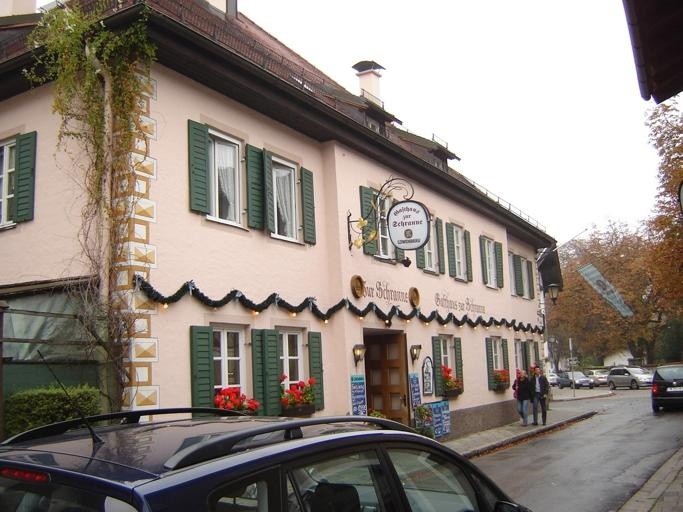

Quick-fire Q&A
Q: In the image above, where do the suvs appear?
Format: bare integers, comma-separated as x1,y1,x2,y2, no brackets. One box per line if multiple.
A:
0,347,536,512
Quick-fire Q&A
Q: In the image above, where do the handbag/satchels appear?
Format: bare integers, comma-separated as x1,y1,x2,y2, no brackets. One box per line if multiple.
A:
513,389,519,399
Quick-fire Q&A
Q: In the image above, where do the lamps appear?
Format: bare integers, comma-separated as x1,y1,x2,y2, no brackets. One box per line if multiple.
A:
545,280,567,306
353,345,367,367
409,345,423,366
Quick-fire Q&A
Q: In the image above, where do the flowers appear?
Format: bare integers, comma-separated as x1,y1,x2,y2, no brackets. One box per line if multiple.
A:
415,403,433,436
441,364,466,391
214,386,261,411
495,368,510,381
277,372,319,405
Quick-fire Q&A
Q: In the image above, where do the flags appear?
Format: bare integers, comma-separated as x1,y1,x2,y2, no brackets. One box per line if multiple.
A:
578,262,634,320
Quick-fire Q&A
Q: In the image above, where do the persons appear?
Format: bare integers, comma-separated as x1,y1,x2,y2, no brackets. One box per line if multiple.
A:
512,370,531,428
528,365,550,426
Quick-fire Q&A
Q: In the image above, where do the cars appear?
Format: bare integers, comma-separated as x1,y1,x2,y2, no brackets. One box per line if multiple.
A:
549,366,654,390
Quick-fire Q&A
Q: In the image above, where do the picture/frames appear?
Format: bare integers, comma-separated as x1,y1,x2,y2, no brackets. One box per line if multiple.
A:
421,356,434,396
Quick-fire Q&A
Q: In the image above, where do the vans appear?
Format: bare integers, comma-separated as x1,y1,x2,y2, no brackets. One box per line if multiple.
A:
651,364,683,414
